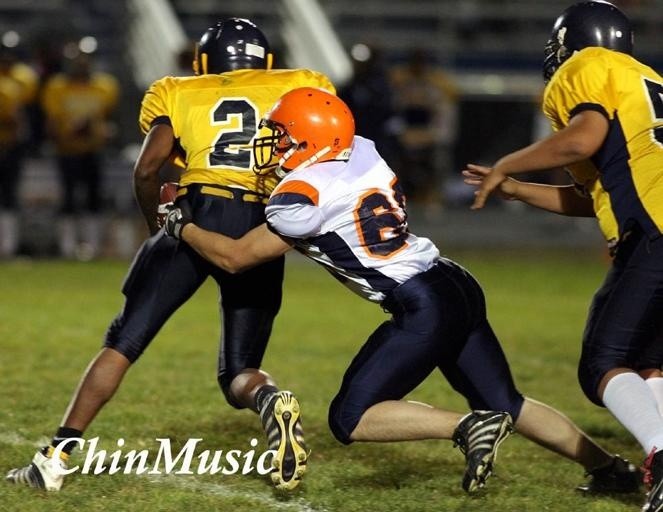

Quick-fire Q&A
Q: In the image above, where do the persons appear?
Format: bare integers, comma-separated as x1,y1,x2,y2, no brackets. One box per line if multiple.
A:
4,16,339,491
459,1,662,511
159,86,649,495
0,27,459,262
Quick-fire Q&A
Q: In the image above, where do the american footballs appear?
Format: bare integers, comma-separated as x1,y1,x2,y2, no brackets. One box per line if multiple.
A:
156,181,178,232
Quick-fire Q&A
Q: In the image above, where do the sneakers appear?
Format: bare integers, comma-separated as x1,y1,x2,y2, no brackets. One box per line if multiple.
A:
452,406,519,493
573,453,639,499
5,449,71,493
638,452,662,512
261,389,312,494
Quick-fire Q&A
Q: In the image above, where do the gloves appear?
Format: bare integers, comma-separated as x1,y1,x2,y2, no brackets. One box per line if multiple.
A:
165,202,193,241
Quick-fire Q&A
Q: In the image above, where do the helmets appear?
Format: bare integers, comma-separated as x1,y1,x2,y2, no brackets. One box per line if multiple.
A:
252,86,356,179
191,15,274,75
541,0,634,86
